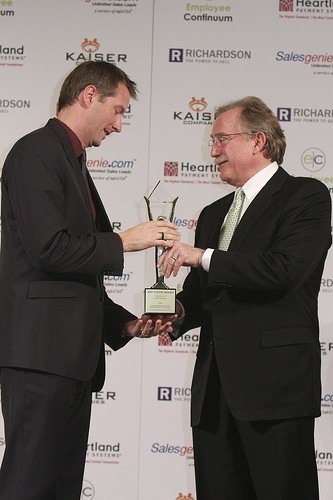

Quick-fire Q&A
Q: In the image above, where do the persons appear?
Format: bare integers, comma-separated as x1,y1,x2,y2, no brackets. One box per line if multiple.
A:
0,61,180,500
142,96,333,500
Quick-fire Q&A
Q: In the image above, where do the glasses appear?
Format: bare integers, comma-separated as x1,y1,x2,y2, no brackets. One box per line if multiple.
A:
209,131,257,147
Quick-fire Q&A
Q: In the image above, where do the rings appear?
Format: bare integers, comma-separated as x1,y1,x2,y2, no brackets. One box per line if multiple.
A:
142,331,148,336
162,232,164,240
172,257,176,260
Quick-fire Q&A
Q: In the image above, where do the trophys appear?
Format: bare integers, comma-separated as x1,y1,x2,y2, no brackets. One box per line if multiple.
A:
143,180,179,315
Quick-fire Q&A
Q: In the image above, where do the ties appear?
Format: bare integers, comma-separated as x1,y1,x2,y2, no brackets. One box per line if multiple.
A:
218,188,246,252
79,150,87,184
